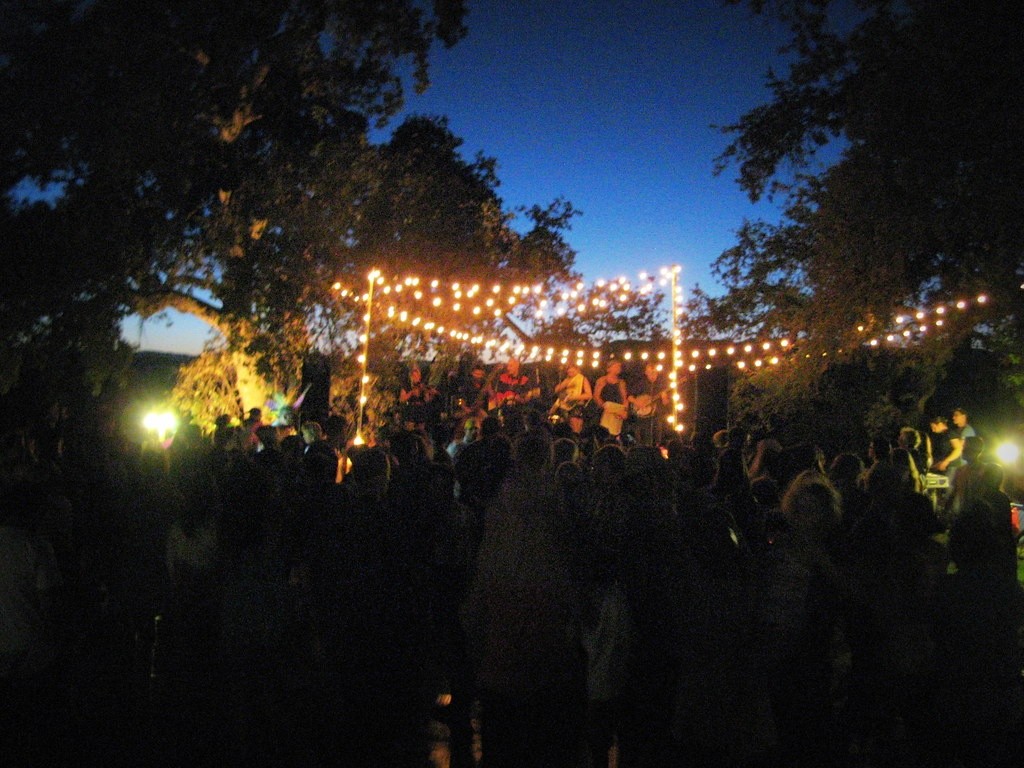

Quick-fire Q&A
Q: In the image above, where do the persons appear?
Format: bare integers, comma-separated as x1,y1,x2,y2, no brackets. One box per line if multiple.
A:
2,320,1024,768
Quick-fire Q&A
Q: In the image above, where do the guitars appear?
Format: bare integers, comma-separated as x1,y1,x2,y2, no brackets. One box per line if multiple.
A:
631,374,688,421
